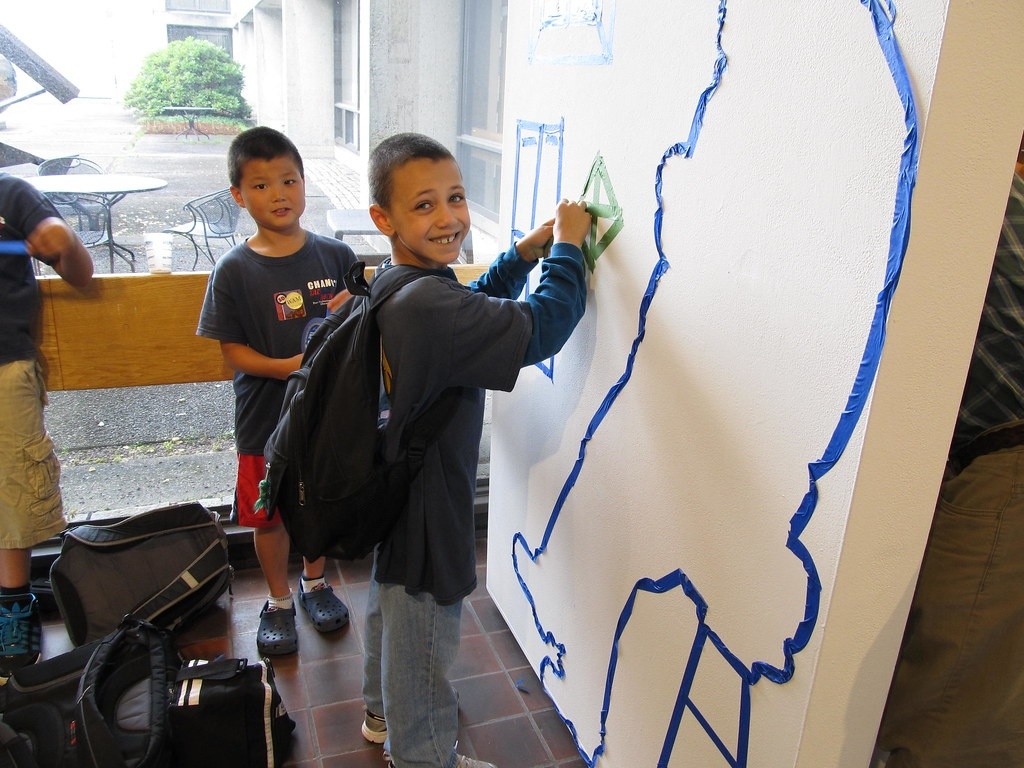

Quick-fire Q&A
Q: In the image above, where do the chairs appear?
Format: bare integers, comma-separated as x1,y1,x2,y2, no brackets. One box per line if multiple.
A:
161,188,242,271
37,192,117,274
37,157,108,232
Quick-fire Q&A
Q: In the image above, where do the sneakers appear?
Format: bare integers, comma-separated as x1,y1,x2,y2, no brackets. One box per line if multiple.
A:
383,740,498,768
0,593,43,686
360,685,461,744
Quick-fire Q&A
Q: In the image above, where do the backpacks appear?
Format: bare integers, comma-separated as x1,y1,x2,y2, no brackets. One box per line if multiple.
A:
49,498,235,635
253,260,433,562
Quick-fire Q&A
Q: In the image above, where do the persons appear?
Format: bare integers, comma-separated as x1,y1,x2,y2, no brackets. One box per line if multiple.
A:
0,173,93,686
196,126,359,654
362,134,592,768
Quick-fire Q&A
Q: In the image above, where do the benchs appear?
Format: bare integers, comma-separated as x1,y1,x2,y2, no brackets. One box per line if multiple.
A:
326,208,473,265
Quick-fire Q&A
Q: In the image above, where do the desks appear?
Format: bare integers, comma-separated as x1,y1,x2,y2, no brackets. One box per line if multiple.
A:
15,174,168,273
163,107,217,141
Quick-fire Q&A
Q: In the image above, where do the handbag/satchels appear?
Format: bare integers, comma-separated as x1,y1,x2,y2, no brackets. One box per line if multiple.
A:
0,624,249,768
163,650,296,768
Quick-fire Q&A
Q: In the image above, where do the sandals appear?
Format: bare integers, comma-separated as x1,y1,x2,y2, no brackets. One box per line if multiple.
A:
255,600,299,655
296,576,350,633
